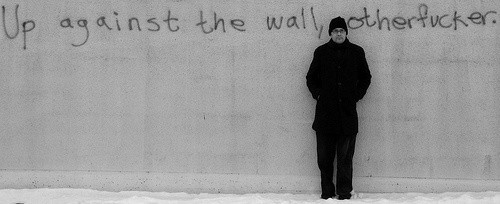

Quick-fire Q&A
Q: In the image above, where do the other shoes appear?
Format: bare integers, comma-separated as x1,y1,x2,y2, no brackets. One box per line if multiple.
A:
319,194,335,199
338,193,351,201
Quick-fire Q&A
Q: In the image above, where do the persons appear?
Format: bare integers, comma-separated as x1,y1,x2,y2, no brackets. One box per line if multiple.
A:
305,16,373,200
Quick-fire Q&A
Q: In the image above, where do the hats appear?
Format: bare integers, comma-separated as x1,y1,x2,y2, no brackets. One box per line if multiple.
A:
328,16,348,35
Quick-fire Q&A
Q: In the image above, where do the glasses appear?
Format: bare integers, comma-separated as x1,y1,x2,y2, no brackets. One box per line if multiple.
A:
331,29,346,34
